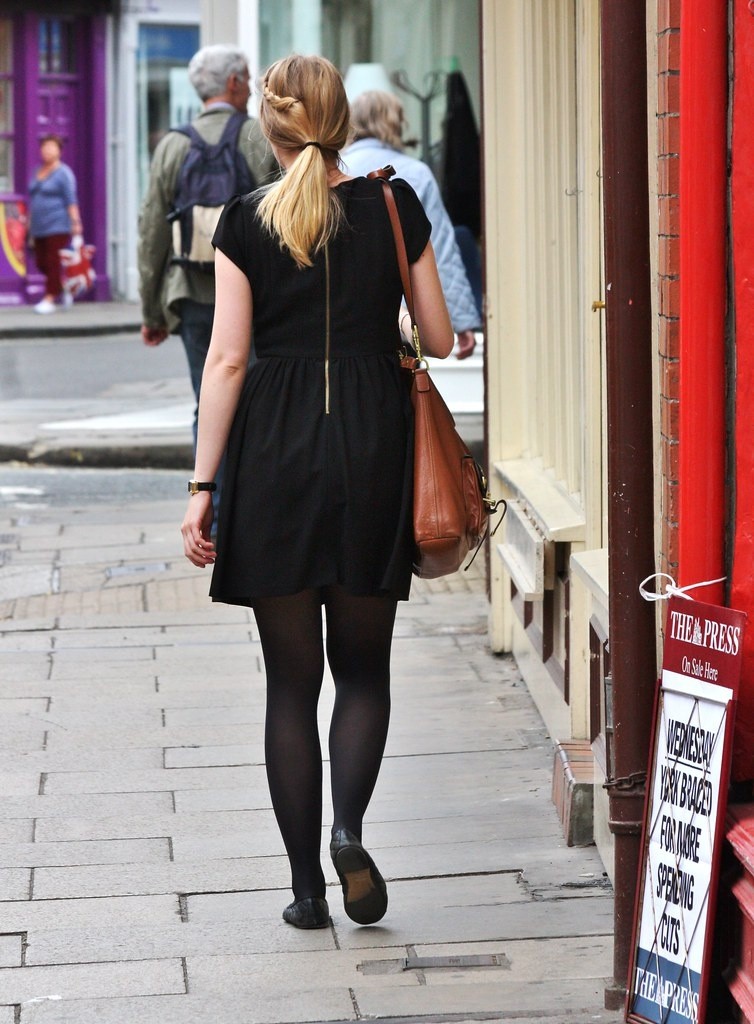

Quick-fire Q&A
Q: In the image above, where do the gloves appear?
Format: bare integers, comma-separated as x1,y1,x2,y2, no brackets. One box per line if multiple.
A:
70,236,84,250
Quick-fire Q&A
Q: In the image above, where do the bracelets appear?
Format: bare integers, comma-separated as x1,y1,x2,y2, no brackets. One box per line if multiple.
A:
187,480,217,496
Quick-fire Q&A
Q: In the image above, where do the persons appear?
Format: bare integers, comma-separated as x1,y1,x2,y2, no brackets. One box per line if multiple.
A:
133,43,287,551
28,133,83,314
337,90,483,361
180,55,455,929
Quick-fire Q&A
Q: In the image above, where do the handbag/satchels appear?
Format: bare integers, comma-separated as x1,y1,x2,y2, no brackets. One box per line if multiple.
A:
368,165,508,579
58,243,98,298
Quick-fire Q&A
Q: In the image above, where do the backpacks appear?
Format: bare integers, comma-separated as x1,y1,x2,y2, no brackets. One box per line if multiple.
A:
170,112,258,272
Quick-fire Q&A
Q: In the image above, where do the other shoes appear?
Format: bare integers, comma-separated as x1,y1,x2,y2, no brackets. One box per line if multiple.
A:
32,300,55,315
283,895,329,929
329,828,388,924
62,293,74,310
